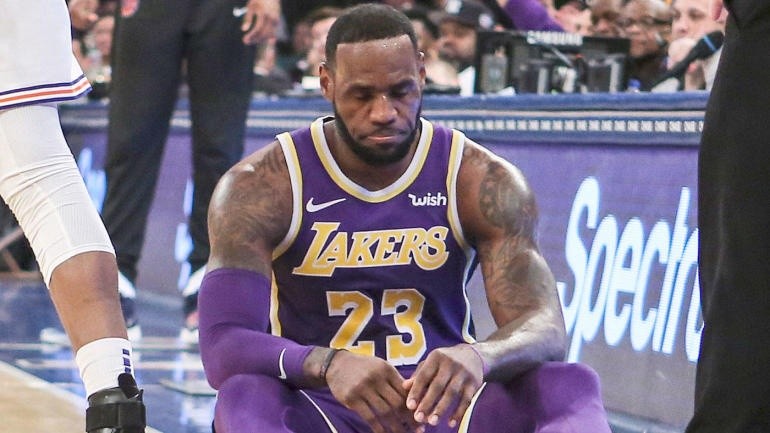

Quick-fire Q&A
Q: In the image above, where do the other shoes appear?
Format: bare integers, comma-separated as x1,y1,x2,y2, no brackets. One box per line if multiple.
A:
85,373,146,433
178,300,201,341
39,290,142,341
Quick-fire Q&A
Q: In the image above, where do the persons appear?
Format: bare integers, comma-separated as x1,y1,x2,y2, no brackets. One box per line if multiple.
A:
197,5,615,432
685,0,770,433
40,0,282,353
68,0,730,97
0,0,148,433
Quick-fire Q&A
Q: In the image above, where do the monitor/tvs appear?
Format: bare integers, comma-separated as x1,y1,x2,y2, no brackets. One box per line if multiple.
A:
473,31,631,95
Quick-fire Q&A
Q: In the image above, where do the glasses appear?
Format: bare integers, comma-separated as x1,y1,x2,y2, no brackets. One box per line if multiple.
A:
620,15,672,30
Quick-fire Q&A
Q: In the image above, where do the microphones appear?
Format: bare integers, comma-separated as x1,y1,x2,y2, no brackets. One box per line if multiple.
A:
648,31,724,91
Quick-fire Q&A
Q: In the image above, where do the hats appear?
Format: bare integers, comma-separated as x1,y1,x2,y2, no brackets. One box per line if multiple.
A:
427,0,494,33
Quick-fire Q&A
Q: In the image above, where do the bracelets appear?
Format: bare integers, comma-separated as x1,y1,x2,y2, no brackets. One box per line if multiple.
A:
318,349,349,384
469,345,490,377
